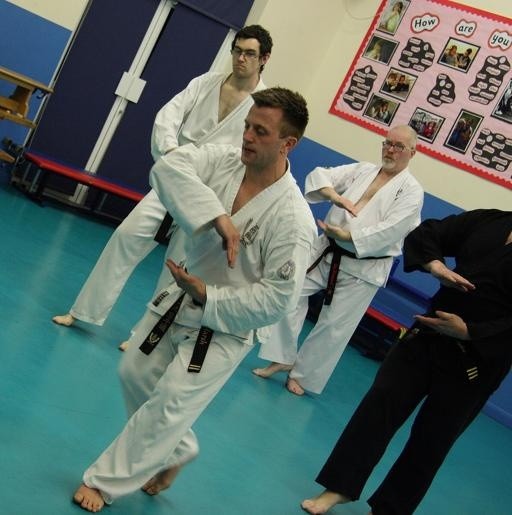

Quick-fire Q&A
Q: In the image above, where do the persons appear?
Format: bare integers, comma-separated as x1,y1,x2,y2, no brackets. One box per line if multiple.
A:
366,41,381,61
300,207,512,513
382,72,410,96
411,120,435,138
448,119,475,149
52,25,272,352
366,100,390,121
71,89,319,513
254,126,424,395
377,2,404,33
444,44,472,70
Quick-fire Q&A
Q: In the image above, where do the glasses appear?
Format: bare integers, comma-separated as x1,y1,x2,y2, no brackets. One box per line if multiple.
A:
383,141,405,154
230,48,261,59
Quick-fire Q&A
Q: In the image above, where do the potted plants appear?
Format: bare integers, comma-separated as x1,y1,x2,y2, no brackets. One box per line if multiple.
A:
0,137,23,164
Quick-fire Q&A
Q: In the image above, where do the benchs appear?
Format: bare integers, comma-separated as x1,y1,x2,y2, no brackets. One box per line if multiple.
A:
11,147,146,226
355,307,410,360
